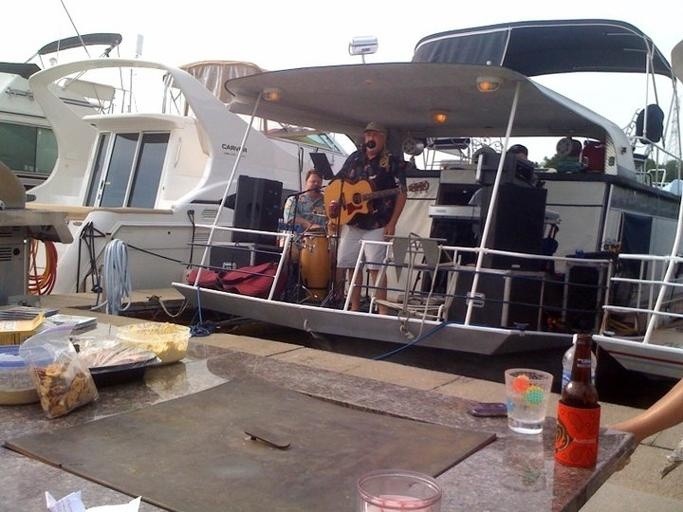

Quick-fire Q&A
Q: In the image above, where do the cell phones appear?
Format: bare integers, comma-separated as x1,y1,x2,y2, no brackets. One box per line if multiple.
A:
470,403,507,417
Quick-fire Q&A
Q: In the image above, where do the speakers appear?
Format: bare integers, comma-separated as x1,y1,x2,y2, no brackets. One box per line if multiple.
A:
230,175,282,246
475,185,548,271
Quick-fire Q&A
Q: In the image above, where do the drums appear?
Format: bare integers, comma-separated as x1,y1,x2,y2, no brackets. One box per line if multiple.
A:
299,231,331,302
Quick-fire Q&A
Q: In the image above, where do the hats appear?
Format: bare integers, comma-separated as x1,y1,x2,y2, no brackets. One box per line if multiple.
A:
362,121,388,133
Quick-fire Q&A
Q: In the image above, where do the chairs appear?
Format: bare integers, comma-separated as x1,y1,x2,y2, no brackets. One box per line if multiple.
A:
425,135,470,170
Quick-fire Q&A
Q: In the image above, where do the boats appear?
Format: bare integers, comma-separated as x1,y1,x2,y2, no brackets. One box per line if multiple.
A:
591,40,682,386
0,32,143,294
170,17,682,377
23,36,377,296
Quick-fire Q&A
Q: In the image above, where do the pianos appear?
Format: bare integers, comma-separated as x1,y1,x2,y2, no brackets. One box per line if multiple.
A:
427,205,561,225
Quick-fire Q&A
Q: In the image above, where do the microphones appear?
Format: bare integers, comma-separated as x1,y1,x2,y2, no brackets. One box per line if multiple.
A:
356,141,376,148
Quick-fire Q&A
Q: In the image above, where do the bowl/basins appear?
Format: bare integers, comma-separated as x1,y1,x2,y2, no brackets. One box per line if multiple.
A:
114,321,192,368
0,345,55,406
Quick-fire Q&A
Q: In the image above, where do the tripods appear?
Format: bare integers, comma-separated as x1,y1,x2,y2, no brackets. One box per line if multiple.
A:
319,154,370,310
287,245,316,302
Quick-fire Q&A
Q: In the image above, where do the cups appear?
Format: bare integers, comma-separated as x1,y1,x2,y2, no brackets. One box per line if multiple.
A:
356,468,442,512
504,367,553,436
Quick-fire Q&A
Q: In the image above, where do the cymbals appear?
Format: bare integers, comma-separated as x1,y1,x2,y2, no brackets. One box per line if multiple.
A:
304,212,328,218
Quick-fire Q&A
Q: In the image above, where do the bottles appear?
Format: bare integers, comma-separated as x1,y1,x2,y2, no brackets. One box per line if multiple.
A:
554,331,600,470
560,332,597,401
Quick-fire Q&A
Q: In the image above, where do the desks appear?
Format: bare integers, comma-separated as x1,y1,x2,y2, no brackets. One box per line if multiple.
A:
0,309,642,511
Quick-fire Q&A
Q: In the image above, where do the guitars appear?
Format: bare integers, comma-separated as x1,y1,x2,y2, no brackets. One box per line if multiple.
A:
324,178,429,226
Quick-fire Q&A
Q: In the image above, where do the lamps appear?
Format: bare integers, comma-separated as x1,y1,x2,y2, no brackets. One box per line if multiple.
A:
346,37,379,64
260,84,284,102
431,110,449,125
475,76,503,94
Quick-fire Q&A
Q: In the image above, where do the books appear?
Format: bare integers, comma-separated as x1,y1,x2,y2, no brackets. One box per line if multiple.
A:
46,315,97,330
0,305,58,321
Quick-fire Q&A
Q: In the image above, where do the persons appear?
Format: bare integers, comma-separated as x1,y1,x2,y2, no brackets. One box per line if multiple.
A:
326,120,408,314
556,129,581,174
506,144,528,161
278,169,330,265
605,379,682,443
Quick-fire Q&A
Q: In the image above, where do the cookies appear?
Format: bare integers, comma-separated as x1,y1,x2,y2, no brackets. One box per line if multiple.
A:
31,363,95,419
